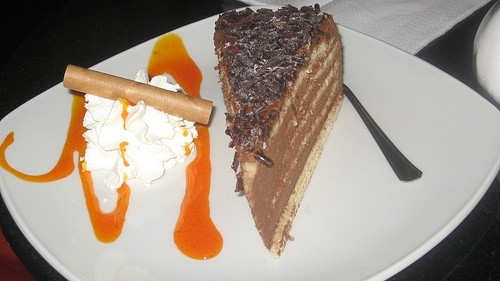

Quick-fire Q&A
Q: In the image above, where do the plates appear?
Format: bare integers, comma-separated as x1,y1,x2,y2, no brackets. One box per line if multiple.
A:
0,5,496,281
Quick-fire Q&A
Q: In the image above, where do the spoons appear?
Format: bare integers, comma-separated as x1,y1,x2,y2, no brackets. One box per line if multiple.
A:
342,83,423,183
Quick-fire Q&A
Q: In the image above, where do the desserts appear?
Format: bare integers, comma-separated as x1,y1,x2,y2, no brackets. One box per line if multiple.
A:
213,4,345,256
